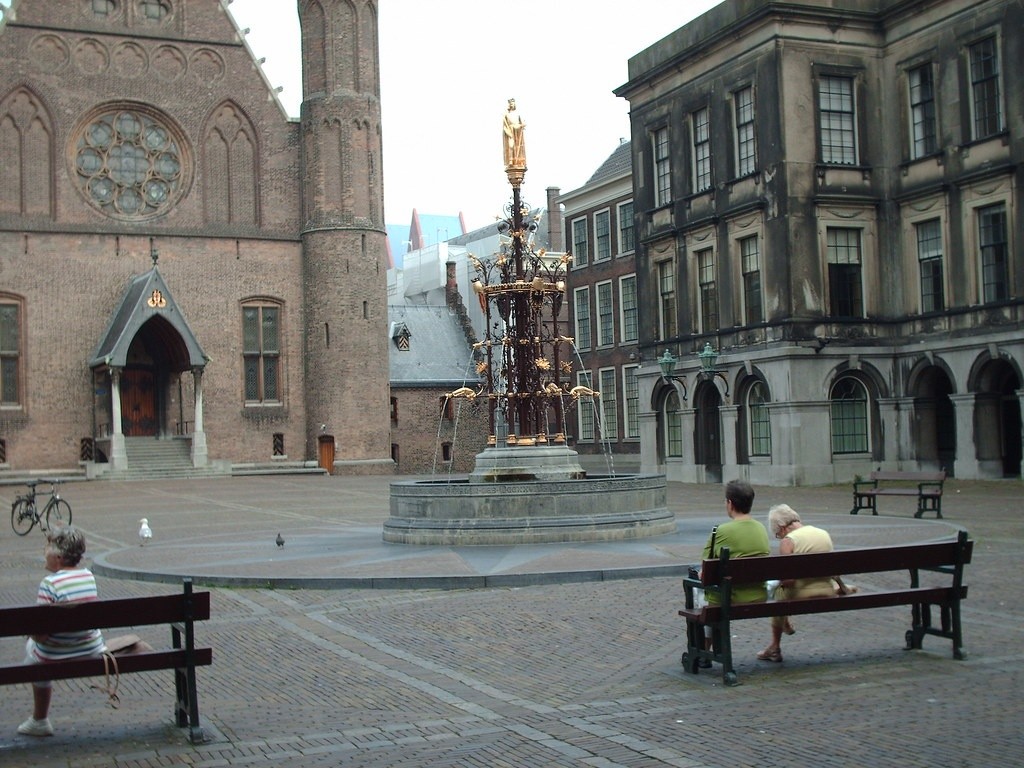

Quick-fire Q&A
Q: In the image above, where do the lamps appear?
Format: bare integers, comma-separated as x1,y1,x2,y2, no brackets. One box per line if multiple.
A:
698,341,730,400
659,349,688,401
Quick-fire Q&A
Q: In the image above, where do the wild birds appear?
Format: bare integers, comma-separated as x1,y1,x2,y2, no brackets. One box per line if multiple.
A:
138,517,153,547
275,532,286,550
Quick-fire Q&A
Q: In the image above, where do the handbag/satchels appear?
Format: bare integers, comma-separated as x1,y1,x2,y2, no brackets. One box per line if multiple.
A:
688,566,699,581
100,633,142,656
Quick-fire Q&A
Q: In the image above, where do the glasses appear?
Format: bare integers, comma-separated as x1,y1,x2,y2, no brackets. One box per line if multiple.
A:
775,533,782,539
722,498,728,503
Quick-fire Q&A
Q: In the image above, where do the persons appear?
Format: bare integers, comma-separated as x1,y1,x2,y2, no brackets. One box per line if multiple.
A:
502,99,526,167
691,479,770,668
17,526,104,736
757,503,836,662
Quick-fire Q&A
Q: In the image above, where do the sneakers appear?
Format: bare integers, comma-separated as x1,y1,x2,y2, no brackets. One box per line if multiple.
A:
16,716,54,736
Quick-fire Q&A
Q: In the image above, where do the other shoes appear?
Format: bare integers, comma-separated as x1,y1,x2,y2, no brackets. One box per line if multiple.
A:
699,657,713,668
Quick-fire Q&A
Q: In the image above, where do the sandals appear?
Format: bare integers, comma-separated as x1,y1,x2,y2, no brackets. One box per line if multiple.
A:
783,621,796,636
756,644,783,662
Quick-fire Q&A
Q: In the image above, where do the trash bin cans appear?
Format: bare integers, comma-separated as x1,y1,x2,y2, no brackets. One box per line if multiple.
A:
318,435,334,474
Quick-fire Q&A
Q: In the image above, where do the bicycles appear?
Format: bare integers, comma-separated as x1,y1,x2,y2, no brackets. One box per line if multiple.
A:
11,478,72,536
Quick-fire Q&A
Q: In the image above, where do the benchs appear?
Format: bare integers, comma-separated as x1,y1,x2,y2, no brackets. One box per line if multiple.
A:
850,466,947,519
678,528,973,687
0,578,213,745
